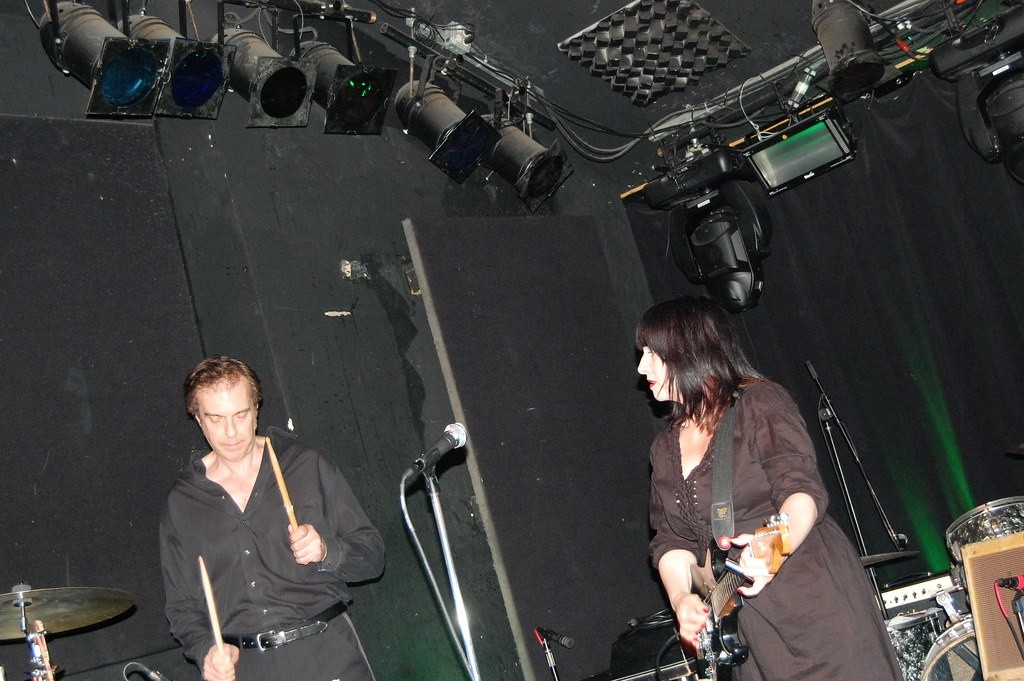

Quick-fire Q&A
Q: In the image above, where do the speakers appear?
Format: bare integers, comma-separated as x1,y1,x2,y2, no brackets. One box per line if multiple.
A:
961,531,1024,681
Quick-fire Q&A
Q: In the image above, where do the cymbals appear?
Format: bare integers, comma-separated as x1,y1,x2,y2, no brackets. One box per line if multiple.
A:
860,550,922,567
0,586,137,640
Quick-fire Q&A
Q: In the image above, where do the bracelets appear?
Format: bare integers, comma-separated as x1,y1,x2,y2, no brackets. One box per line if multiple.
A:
317,539,326,563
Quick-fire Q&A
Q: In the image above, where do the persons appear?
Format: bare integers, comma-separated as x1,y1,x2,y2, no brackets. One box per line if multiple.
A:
635,296,903,681
157,356,385,681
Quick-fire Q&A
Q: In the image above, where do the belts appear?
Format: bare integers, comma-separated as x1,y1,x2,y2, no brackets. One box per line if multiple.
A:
224,600,348,651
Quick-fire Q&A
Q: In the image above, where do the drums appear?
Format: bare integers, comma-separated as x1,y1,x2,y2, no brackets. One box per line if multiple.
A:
884,607,945,681
945,495,1024,605
920,618,985,681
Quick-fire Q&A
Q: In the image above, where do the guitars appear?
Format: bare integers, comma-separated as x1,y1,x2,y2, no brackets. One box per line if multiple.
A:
689,512,791,681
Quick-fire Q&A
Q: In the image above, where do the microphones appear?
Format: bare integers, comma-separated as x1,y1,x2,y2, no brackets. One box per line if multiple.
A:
402,423,469,479
138,662,169,681
995,575,1024,591
898,534,908,552
536,626,575,648
